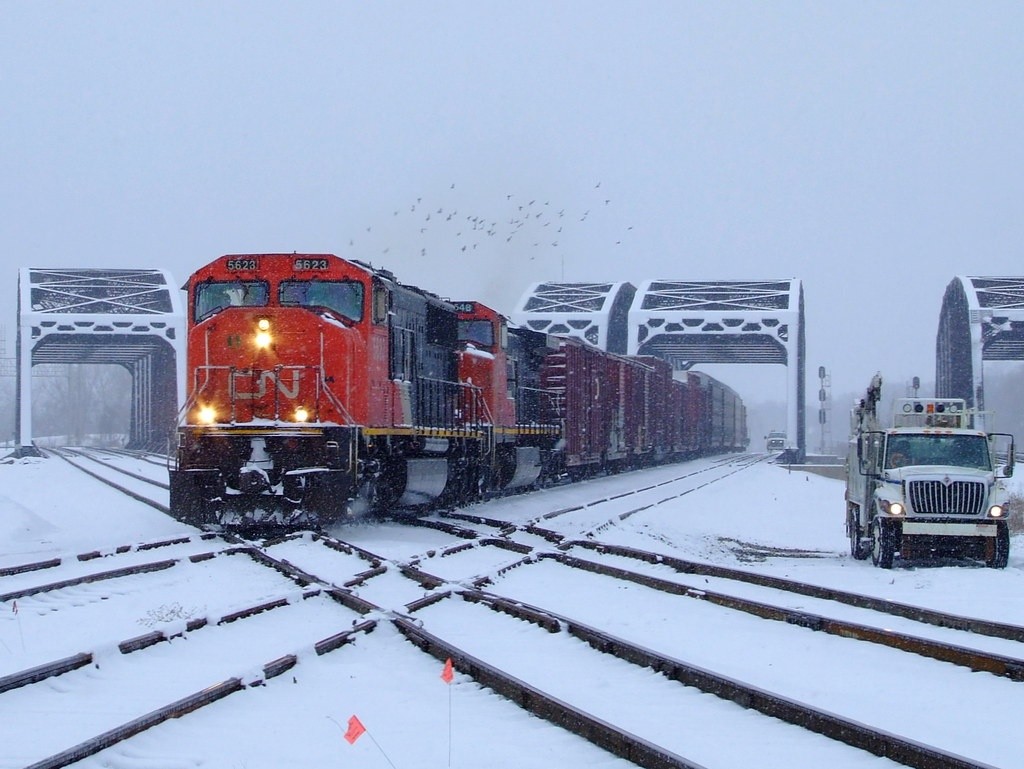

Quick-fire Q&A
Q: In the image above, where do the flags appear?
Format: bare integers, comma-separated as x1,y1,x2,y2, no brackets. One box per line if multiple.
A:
440,659,453,683
344,716,365,745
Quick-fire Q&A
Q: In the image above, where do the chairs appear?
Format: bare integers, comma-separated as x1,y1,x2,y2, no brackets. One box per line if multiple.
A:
890,441,915,468
950,438,974,465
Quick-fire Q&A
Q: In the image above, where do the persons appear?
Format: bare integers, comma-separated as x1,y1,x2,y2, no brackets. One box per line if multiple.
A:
217,293,231,307
888,441,918,467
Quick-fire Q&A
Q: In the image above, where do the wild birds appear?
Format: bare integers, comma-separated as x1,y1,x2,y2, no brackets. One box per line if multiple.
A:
349,182,633,261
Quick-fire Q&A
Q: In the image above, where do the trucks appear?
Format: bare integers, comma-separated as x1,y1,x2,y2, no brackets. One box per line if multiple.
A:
764,430,788,453
843,398,1017,572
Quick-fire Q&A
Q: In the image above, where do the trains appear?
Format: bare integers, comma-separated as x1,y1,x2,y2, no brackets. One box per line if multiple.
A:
168,251,750,543
